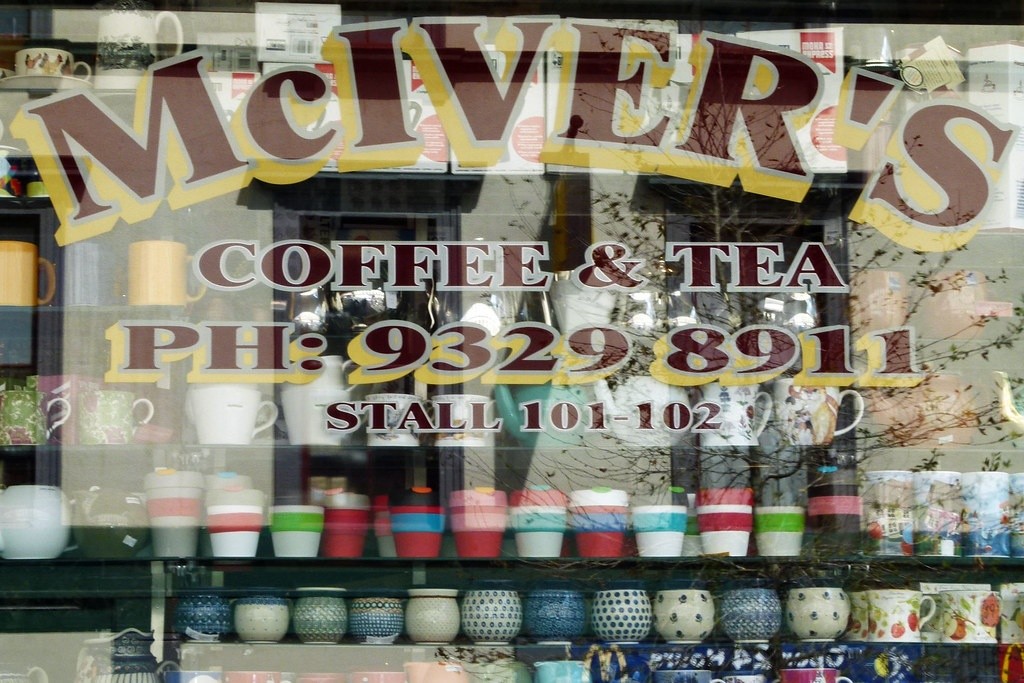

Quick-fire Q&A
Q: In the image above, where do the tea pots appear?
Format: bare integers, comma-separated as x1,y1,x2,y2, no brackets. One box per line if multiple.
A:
493,376,692,448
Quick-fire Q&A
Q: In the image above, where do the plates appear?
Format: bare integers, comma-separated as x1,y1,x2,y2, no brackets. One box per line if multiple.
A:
0,74,94,90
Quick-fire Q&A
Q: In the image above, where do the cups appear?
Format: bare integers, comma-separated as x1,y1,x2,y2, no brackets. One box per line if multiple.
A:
162,660,855,683
0,11,184,90
698,379,865,446
0,240,208,305
0,468,1024,557
0,145,61,197
177,587,1024,642
849,271,991,339
550,270,618,337
0,355,493,447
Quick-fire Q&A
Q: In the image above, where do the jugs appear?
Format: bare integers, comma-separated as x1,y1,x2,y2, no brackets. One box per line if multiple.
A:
865,369,1024,446
